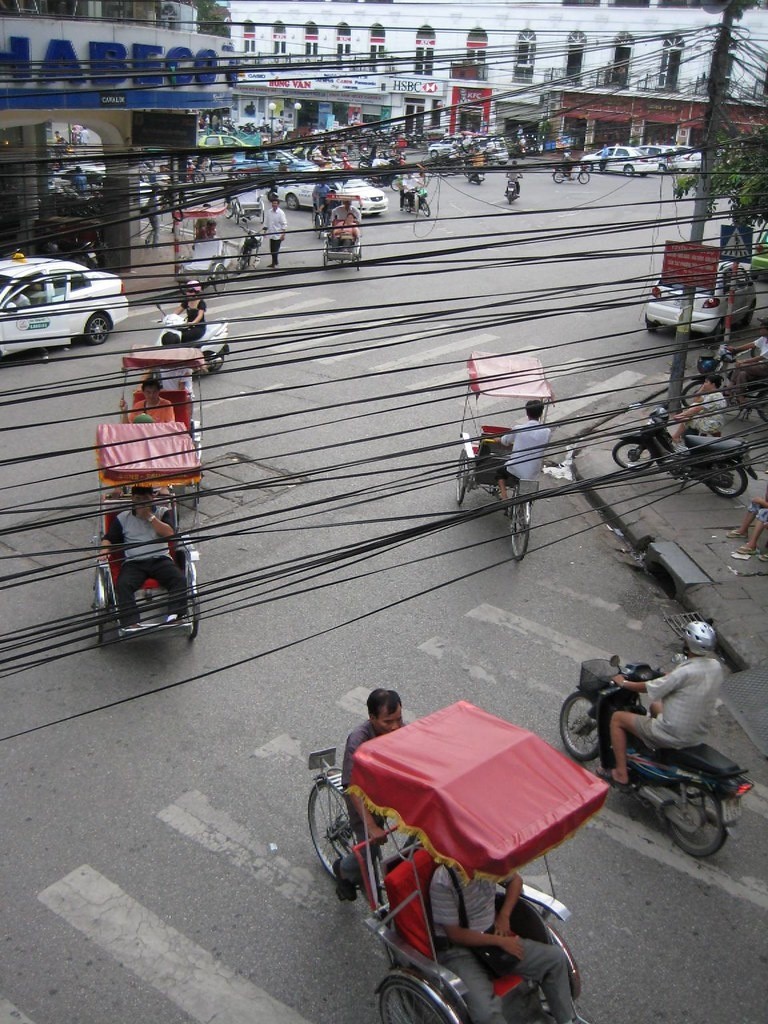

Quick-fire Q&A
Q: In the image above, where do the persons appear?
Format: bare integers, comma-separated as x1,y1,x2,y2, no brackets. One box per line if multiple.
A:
430,864,574,1024
192,203,217,250
332,213,361,253
199,113,219,132
145,161,196,187
667,373,728,443
596,620,725,794
9,287,49,359
618,64,626,85
726,480,768,554
145,192,162,248
133,332,194,420
333,688,403,900
493,400,552,504
119,378,175,424
96,487,188,633
53,131,70,170
69,165,87,196
724,316,768,403
596,143,613,171
263,200,287,267
172,280,207,342
300,124,579,221
260,116,283,139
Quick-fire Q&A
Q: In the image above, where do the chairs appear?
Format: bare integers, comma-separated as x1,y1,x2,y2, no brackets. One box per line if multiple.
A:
102,499,195,632
330,219,357,249
182,236,222,274
132,388,190,430
472,426,513,459
383,846,556,1004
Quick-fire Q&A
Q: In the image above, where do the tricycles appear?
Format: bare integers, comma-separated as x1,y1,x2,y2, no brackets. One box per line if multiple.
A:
307,702,612,1024
121,344,204,506
311,192,364,271
92,422,202,647
174,205,228,297
457,349,557,562
224,178,266,224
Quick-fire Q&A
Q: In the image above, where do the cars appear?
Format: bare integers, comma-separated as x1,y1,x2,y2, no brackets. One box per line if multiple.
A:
231,149,321,180
198,133,257,159
277,166,389,220
578,145,667,177
0,253,129,360
638,144,703,172
426,136,509,166
645,259,757,345
749,223,768,281
140,169,171,212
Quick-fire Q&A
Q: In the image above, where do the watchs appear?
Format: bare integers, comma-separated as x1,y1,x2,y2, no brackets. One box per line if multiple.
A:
148,514,155,522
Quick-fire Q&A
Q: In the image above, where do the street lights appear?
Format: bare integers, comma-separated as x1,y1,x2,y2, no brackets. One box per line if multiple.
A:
294,103,301,132
268,103,276,144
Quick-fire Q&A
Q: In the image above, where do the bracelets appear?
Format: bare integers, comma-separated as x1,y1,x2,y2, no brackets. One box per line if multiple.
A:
620,680,628,688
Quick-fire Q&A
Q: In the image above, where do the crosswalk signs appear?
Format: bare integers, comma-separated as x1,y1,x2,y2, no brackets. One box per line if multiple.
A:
719,224,752,264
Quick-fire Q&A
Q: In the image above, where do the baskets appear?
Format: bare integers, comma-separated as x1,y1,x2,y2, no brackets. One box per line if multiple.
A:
580,659,622,703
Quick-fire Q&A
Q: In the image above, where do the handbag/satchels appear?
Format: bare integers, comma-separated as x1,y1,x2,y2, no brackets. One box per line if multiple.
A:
140,206,150,214
471,924,520,978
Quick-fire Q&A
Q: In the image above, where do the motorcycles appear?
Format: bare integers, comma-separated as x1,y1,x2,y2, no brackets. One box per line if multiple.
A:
682,340,768,424
610,400,758,499
560,654,755,859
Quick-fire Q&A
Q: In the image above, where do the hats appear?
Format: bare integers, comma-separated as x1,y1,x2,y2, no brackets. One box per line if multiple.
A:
757,316,768,324
133,413,154,423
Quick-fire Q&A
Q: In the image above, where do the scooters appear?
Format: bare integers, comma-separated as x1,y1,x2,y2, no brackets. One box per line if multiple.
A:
32,216,97,269
187,155,224,185
517,134,545,160
235,216,269,275
293,140,431,217
552,162,591,185
155,301,231,375
504,176,524,205
433,147,488,185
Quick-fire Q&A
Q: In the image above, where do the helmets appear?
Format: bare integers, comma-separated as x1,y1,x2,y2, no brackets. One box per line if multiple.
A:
697,355,717,374
186,280,202,294
684,622,717,656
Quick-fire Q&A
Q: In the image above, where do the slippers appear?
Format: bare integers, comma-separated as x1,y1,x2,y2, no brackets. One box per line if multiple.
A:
727,530,747,537
737,545,757,554
759,554,768,561
597,767,631,792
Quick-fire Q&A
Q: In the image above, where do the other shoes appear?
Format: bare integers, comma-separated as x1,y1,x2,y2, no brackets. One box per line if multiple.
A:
145,239,149,245
170,620,184,624
400,208,403,211
267,264,277,268
407,208,411,212
332,858,358,901
125,624,140,629
672,438,682,444
